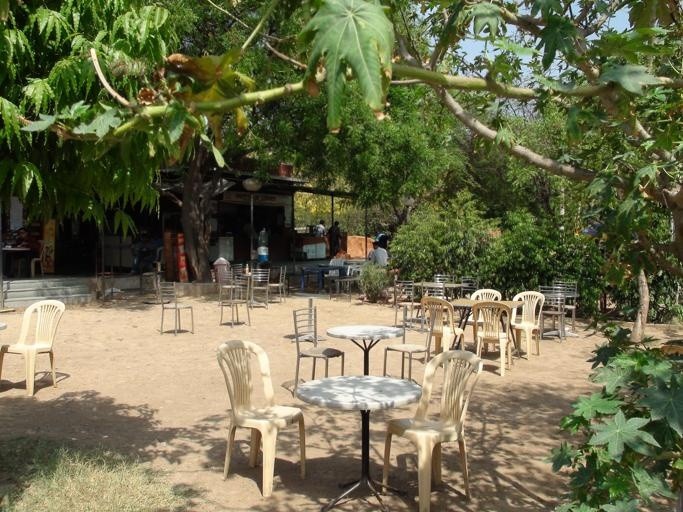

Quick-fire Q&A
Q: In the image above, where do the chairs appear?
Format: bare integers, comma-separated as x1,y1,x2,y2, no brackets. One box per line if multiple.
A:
214,339,306,499
394,274,581,376
17,241,46,278
151,247,164,273
0,298,67,397
293,259,366,301
158,281,194,336
215,262,287,331
379,348,483,511
381,306,436,382
292,304,344,386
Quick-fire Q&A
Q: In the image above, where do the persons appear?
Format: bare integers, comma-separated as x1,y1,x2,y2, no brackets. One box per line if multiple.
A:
369,241,388,267
314,219,328,242
328,221,342,255
16,228,41,276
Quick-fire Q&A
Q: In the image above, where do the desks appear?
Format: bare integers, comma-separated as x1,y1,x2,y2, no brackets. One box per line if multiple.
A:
302,234,374,257
0,246,30,278
292,374,423,511
326,323,404,375
